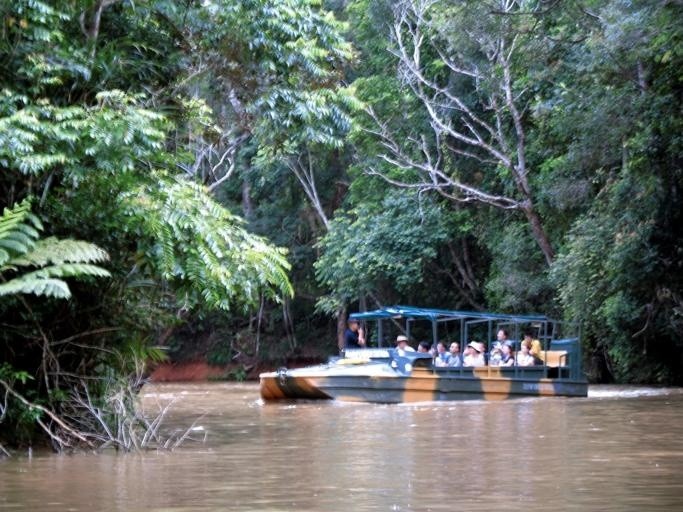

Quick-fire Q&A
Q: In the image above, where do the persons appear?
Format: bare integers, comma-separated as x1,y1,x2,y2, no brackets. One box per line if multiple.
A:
344,318,366,348
396,329,544,368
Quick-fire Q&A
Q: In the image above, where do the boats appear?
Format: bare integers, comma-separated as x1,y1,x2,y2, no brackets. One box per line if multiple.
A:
258,303,590,406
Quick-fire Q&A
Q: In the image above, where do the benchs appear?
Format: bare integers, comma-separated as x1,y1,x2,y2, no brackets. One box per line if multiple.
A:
512,350,567,368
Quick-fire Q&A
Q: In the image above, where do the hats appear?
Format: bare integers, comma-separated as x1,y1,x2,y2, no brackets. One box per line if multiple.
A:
468,341,482,352
397,335,408,342
